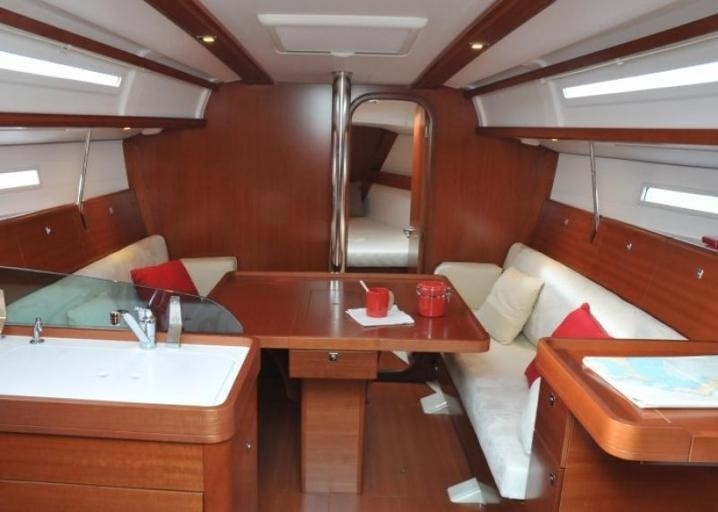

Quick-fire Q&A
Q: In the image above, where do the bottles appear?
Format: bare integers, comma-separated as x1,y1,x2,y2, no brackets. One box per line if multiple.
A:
416,280,449,317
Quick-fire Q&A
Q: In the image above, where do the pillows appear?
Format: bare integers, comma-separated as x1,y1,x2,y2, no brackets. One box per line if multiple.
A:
69,292,122,330
525,303,615,388
474,265,544,343
130,259,205,318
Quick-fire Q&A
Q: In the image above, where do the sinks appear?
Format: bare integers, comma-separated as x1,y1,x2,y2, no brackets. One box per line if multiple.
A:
0,334,251,407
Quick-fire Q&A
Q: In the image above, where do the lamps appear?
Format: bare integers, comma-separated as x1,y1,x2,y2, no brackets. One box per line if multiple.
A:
255,12,430,58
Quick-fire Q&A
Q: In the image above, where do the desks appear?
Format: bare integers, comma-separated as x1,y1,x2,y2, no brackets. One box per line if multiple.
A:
177,269,485,511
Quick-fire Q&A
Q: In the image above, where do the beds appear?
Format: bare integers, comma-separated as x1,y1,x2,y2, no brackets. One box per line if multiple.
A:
345,171,420,267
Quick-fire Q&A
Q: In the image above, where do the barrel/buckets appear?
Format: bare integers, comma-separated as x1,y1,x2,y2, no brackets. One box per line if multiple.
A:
417,278,452,319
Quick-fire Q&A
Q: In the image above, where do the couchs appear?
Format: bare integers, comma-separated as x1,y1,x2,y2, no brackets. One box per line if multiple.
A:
430,242,692,512
1,232,238,338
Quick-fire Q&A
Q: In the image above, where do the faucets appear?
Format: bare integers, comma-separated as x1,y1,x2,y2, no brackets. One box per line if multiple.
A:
110,305,157,350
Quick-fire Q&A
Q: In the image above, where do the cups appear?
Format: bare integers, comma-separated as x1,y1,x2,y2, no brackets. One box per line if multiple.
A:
366,287,394,317
